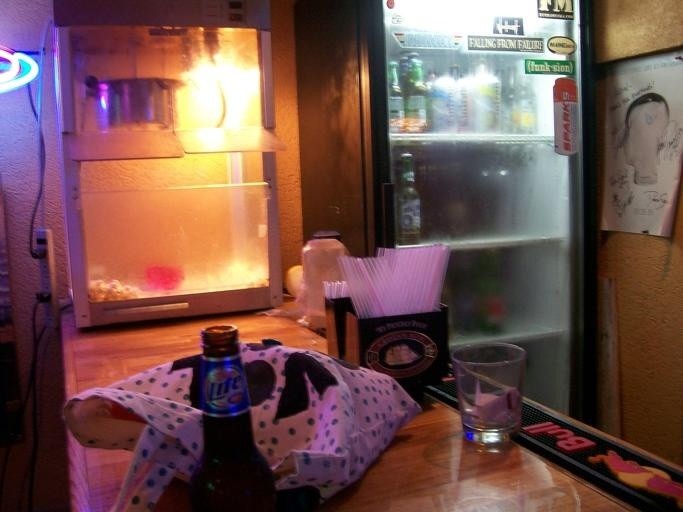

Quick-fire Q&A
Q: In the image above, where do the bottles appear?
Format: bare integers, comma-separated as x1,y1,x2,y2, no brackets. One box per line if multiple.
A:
450,242,541,339
391,139,541,240
382,46,538,138
187,325,276,512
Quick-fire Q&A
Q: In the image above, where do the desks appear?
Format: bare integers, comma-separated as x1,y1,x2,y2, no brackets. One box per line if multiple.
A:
57,309,682,512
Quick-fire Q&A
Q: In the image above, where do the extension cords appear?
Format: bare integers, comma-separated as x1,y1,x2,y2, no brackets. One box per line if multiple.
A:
35,229,63,356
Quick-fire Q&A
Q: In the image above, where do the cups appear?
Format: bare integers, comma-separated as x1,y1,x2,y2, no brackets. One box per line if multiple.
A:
452,342,527,444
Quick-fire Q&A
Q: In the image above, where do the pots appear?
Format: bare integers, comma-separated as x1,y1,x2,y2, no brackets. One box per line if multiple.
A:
95,77,183,135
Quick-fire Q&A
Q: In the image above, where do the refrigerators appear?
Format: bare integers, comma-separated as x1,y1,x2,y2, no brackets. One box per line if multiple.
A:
293,0,598,425
